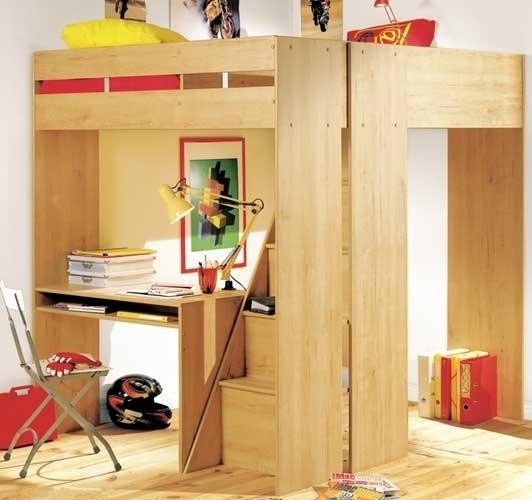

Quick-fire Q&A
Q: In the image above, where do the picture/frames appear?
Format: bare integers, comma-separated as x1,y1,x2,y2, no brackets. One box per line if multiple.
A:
178,135,248,274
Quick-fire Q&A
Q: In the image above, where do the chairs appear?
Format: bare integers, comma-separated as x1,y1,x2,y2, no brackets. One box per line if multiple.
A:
1,286,123,479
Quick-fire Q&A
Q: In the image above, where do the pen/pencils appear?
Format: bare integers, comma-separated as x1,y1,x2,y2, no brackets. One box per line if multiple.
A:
199,255,219,290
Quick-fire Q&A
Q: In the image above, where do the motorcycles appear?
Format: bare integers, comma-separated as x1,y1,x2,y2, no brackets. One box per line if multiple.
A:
204,0,236,39
309,0,331,32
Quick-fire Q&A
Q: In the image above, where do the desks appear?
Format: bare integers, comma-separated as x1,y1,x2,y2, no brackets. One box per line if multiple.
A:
35,282,246,474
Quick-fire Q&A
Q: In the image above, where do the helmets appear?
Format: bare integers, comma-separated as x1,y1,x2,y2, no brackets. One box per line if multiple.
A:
106,374,171,430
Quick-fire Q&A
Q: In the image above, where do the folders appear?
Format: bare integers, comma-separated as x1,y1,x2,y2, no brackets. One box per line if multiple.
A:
418,348,498,426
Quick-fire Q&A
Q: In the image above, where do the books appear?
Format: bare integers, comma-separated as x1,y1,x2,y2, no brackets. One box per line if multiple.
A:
313,473,401,500
55,299,111,314
115,310,179,323
415,347,499,427
66,245,159,288
125,284,197,297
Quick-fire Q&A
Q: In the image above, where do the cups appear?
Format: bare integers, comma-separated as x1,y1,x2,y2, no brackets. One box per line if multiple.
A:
197,267,219,294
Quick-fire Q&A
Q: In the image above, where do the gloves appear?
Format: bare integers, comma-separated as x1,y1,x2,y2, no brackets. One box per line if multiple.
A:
46,352,102,377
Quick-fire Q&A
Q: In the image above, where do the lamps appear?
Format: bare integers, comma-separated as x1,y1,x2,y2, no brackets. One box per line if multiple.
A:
157,176,265,294
373,0,408,46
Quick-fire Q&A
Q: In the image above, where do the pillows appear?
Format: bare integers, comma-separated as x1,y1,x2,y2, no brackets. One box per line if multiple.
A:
58,19,192,49
